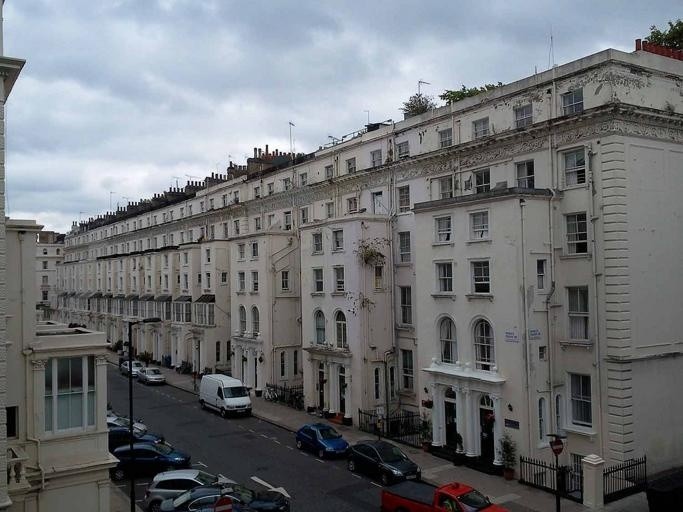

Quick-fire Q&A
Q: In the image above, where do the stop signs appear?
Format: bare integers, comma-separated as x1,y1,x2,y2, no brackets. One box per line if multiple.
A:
214,497,232,512
552,440,563,455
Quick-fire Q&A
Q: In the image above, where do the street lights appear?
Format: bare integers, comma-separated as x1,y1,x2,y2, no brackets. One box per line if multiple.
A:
128,317,163,512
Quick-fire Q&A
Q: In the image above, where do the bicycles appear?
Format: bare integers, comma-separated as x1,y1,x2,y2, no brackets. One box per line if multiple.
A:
264,387,278,402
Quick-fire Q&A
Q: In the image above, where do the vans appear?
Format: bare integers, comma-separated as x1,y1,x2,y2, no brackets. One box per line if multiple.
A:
199,374,253,419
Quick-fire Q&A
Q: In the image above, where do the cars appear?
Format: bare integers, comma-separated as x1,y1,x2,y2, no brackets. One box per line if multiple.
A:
157,485,290,512
120,361,144,378
107,416,148,437
138,367,166,385
346,438,420,483
294,423,348,459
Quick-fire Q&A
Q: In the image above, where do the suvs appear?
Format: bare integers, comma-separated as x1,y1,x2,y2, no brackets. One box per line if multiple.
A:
110,443,191,481
108,426,165,452
143,470,240,512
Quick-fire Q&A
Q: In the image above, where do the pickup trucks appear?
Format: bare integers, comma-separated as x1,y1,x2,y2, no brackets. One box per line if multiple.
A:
380,477,509,512
